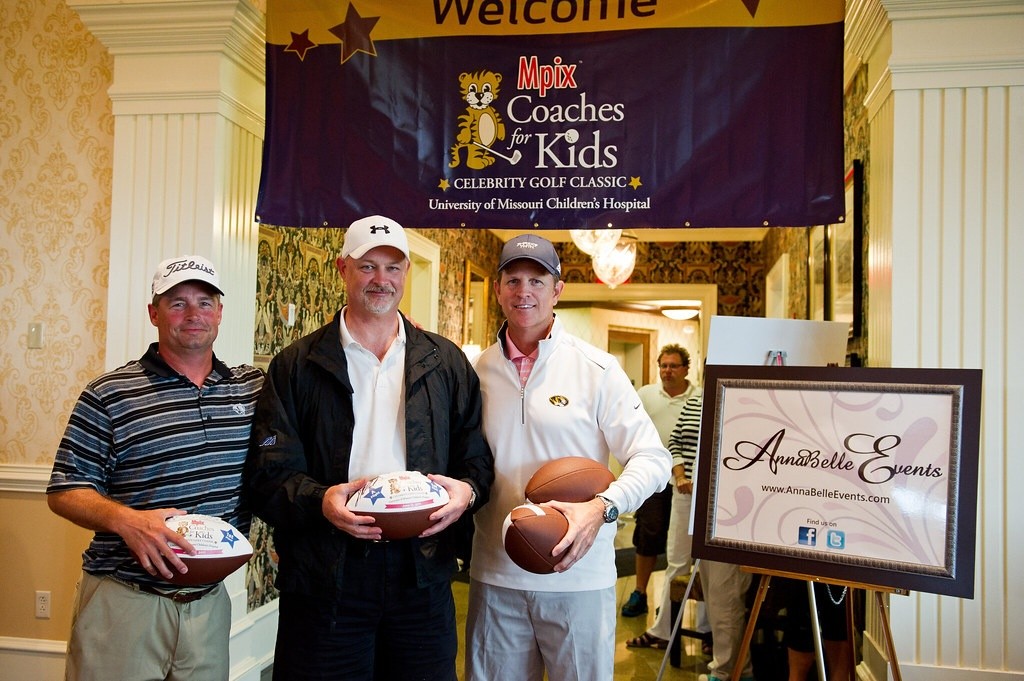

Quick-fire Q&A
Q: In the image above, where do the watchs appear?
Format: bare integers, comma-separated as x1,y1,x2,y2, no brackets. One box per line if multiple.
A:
601,498,619,524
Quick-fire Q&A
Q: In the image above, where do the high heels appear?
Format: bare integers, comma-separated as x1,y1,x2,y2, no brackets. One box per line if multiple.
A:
702,638,714,655
627,631,669,650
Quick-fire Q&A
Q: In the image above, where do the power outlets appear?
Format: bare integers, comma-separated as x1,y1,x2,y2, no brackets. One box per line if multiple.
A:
35,591,51,618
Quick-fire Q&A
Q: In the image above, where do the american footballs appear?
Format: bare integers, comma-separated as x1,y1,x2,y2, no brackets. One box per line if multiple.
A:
500,503,572,575
524,456,617,505
134,513,257,586
347,470,451,540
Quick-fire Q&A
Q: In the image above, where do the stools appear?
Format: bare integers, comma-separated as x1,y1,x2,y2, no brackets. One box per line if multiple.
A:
670,571,712,668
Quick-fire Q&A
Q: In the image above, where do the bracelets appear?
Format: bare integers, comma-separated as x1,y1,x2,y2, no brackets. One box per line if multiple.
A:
466,487,476,510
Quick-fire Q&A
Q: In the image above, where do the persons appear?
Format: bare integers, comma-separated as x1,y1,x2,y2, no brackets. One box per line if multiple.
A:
783,577,856,681
45,254,268,680
242,214,496,681
403,234,673,681
626,356,713,654
620,343,697,618
698,559,752,681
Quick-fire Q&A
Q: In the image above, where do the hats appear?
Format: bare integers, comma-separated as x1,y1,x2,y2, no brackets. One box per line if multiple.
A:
151,254,225,300
343,216,411,263
497,233,561,279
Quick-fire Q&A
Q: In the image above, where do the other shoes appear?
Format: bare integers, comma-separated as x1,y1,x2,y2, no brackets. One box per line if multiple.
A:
621,588,648,616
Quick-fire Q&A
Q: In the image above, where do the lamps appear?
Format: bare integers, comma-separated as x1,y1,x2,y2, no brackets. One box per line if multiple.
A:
569,229,636,289
662,310,698,320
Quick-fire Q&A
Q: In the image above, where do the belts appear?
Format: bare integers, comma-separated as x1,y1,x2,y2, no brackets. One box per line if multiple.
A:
125,576,218,602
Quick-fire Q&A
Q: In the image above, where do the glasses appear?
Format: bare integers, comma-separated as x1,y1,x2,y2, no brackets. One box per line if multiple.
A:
658,362,685,369
